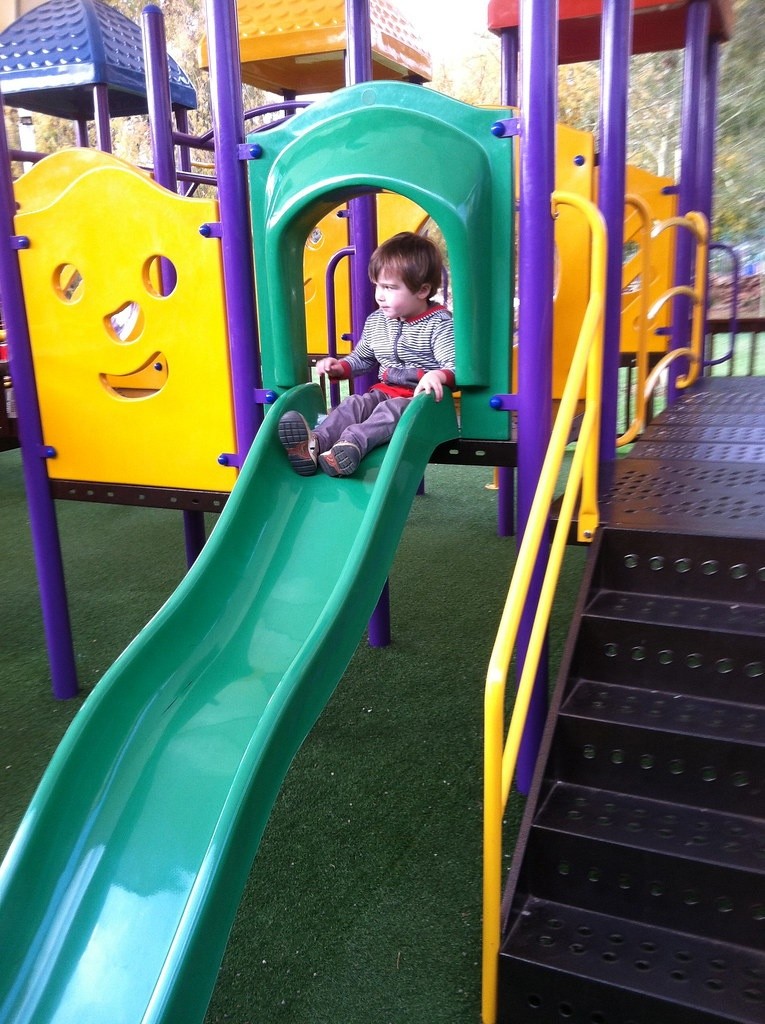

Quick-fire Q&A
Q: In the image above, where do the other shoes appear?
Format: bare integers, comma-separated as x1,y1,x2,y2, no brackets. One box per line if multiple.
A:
318,443,362,476
278,411,319,475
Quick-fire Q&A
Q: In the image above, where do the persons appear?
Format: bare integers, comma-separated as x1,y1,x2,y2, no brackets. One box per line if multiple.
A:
278,231,456,477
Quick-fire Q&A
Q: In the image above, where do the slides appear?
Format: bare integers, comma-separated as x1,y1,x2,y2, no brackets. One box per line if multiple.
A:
0,382,461,1024
